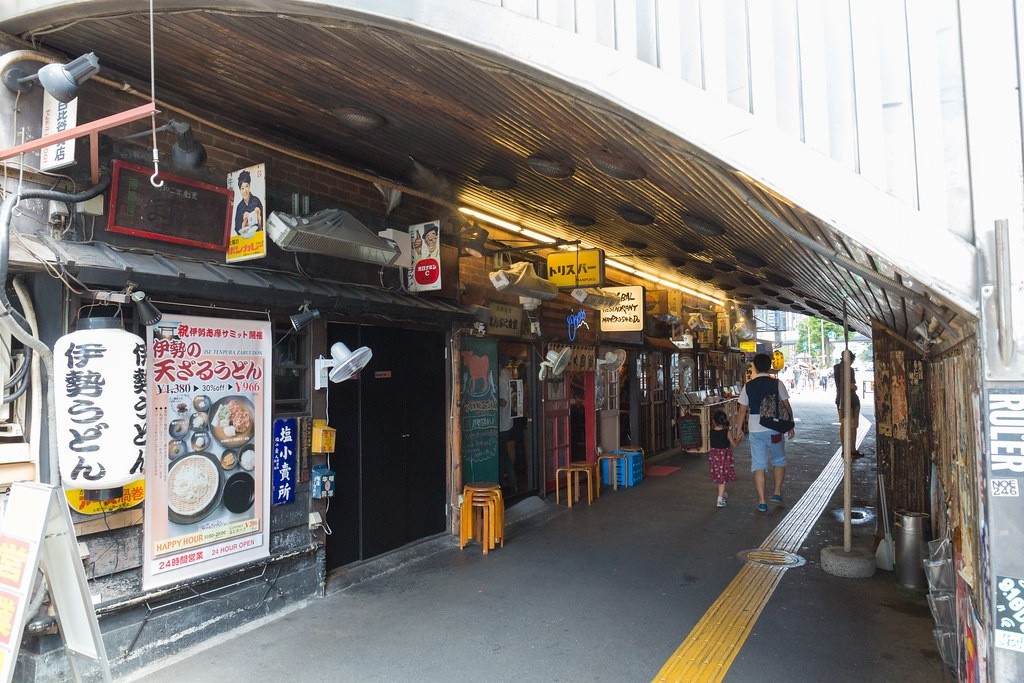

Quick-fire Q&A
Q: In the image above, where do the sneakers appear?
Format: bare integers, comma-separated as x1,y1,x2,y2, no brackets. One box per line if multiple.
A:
771,495,783,504
724,492,728,499
757,503,767,511
716,500,726,506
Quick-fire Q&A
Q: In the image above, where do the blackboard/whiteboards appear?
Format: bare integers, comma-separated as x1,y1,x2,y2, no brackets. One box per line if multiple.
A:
105,160,234,251
460,332,500,520
677,415,702,449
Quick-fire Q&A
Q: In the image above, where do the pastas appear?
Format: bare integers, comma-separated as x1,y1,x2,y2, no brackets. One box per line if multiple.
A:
174,464,212,502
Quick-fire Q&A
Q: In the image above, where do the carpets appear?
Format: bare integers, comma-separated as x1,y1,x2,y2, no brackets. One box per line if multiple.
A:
642,465,682,477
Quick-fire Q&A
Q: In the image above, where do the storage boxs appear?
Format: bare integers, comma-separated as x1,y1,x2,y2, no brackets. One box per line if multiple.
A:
602,449,643,487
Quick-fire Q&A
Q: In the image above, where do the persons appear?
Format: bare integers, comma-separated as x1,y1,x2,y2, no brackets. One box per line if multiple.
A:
411,223,439,266
620,379,631,447
780,360,834,393
234,171,263,232
834,351,865,458
498,352,519,498
736,354,795,511
708,410,744,508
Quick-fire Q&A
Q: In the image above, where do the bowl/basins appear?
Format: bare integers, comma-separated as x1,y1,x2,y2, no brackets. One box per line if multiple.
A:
220,450,239,471
224,472,255,513
239,444,255,471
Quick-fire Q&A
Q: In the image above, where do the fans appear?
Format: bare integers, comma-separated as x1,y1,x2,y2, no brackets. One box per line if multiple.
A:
670,356,698,378
315,341,373,390
596,350,629,372
539,348,575,380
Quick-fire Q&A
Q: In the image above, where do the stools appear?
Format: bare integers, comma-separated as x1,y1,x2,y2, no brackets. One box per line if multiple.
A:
619,445,646,479
556,453,628,509
459,482,505,554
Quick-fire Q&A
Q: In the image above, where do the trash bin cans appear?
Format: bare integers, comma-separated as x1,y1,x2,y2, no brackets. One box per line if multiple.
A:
894,508,931,589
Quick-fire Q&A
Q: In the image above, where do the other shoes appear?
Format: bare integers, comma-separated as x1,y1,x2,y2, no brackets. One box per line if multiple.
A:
841,450,864,457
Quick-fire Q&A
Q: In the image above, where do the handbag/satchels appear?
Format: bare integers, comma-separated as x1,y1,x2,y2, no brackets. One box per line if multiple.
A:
759,379,795,434
791,382,794,388
821,379,825,384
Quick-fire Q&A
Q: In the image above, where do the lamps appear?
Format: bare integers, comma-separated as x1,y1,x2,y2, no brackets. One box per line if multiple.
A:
290,305,320,332
126,292,162,325
97,120,208,169
2,52,99,104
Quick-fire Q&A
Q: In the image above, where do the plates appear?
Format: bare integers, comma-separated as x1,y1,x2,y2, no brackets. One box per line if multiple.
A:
169,420,188,439
189,412,209,431
168,440,187,461
191,430,211,452
168,453,225,524
208,395,255,449
194,395,211,412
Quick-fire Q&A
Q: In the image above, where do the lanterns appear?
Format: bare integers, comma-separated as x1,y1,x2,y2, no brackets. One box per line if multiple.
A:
772,351,784,370
54,315,146,502
